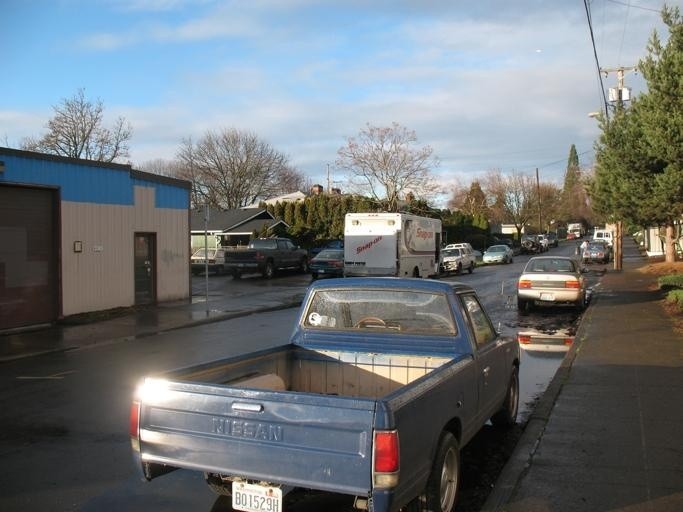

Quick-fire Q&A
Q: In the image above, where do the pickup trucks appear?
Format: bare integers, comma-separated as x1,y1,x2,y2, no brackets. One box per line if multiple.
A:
190,237,308,279
520,233,558,255
130,276,520,511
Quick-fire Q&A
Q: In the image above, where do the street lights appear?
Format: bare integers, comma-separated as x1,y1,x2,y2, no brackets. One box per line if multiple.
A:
193,203,209,300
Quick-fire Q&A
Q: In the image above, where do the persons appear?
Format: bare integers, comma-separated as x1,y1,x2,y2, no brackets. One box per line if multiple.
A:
579,240,589,261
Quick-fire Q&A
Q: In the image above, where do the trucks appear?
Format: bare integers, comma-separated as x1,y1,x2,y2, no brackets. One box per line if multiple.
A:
566,223,585,239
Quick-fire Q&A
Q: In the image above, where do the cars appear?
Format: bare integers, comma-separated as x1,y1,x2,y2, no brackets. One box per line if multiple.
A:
517,256,590,310
306,211,514,279
582,229,613,264
